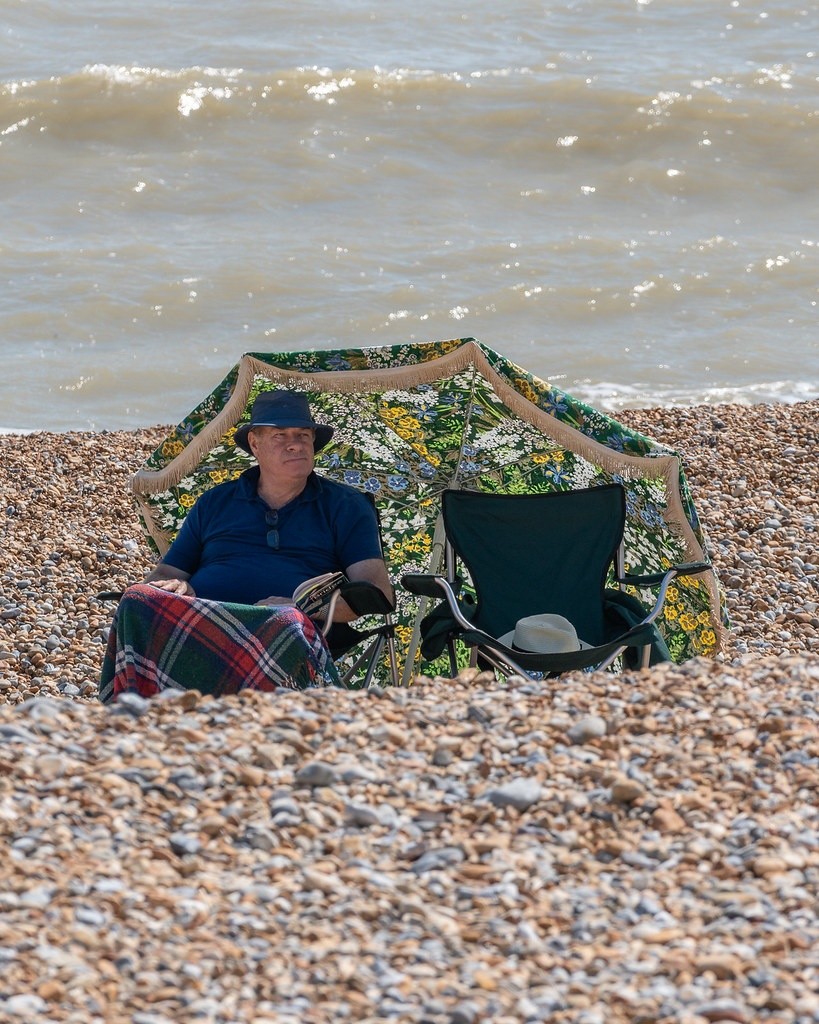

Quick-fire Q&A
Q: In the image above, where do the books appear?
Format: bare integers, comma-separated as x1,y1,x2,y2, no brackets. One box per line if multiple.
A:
263,570,352,623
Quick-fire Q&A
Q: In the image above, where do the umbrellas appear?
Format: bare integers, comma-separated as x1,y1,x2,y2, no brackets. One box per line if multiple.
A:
127,336,724,696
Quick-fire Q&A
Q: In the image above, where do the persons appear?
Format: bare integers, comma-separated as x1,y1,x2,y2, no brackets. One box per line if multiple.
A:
118,390,395,696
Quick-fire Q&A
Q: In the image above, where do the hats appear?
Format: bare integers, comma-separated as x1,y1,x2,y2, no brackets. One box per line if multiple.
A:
495,613,596,654
233,390,334,456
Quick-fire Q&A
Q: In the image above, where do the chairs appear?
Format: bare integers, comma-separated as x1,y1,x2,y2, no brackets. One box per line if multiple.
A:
96,489,414,693
400,480,713,681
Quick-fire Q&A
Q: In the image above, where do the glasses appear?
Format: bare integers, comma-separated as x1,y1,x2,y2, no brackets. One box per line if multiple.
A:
264,507,282,551
